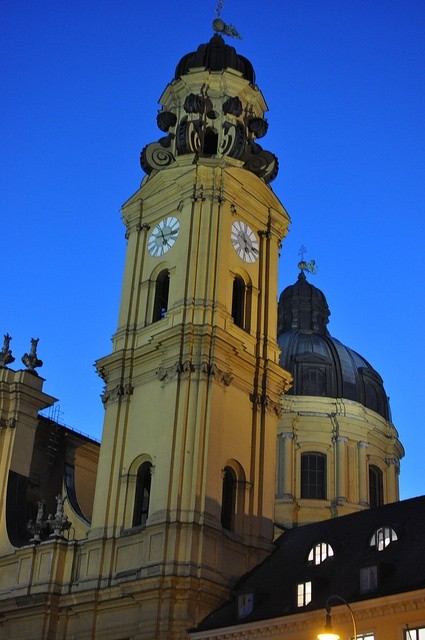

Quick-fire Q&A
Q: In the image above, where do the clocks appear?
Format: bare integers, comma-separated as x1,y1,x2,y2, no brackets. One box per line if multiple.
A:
147,217,180,257
231,220,259,263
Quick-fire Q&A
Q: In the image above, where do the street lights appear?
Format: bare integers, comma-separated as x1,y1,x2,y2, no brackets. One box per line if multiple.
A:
318,595,357,640
